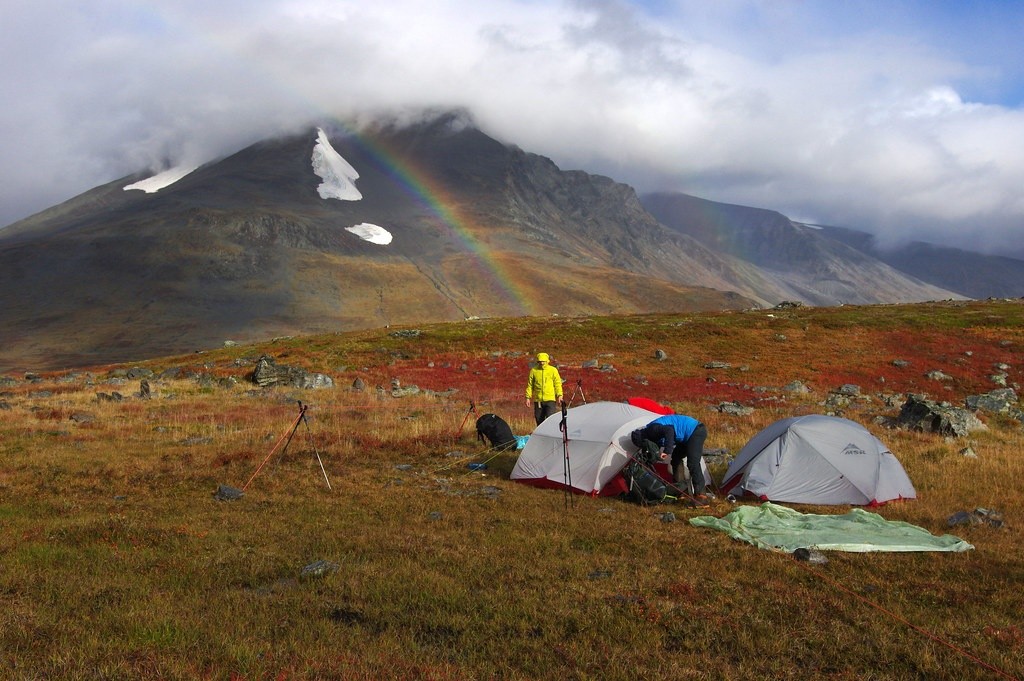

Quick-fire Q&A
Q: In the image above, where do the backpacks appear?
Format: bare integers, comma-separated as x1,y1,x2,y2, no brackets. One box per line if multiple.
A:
623,462,668,504
476,414,517,452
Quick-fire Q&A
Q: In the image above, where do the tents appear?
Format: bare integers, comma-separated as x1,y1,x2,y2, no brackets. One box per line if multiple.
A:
510,400,718,498
719,414,917,509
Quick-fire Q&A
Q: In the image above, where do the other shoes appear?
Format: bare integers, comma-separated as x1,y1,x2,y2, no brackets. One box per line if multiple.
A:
678,493,689,499
693,491,707,499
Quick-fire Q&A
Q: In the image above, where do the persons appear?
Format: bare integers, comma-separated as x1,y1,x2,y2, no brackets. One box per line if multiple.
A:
525,353,563,427
630,414,708,505
622,397,676,416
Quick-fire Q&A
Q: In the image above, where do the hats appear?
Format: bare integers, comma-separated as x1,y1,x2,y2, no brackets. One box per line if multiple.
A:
631,430,644,448
